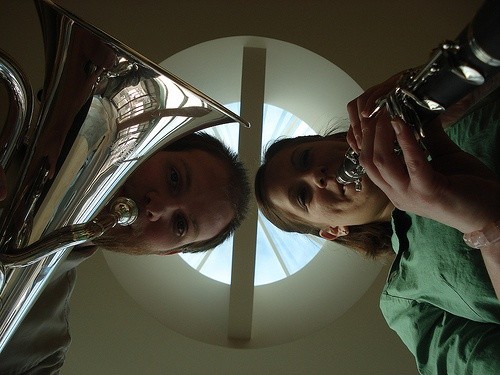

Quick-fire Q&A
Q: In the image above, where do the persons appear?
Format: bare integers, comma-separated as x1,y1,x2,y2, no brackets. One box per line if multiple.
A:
0,67,252,375
253,38,500,374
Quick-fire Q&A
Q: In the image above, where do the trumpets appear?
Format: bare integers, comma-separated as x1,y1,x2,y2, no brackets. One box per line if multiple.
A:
336,0,500,192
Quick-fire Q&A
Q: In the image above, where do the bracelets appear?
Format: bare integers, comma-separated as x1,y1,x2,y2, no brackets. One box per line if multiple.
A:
461,221,500,250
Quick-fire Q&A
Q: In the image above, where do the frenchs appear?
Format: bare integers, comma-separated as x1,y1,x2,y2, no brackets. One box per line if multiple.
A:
1,0,251,355
